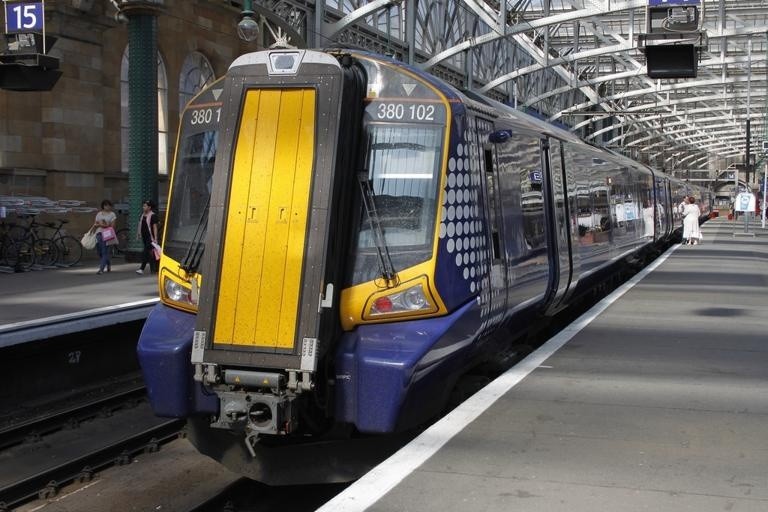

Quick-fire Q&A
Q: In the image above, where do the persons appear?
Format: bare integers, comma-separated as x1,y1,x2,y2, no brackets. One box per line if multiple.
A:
94,200,117,276
682,196,702,247
135,199,162,274
678,195,689,244
643,202,654,237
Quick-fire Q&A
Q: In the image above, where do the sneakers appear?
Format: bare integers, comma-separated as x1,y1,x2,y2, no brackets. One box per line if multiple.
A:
98,268,111,274
136,269,144,275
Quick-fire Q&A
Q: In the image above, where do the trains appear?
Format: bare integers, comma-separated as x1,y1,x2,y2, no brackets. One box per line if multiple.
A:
126,43,717,486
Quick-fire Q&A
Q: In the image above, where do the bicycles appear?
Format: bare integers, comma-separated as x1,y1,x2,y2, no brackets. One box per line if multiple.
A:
0,212,83,272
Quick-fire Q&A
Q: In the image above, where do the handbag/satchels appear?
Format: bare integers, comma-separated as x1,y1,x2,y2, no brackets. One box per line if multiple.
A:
101,223,119,247
80,224,99,251
153,243,161,260
